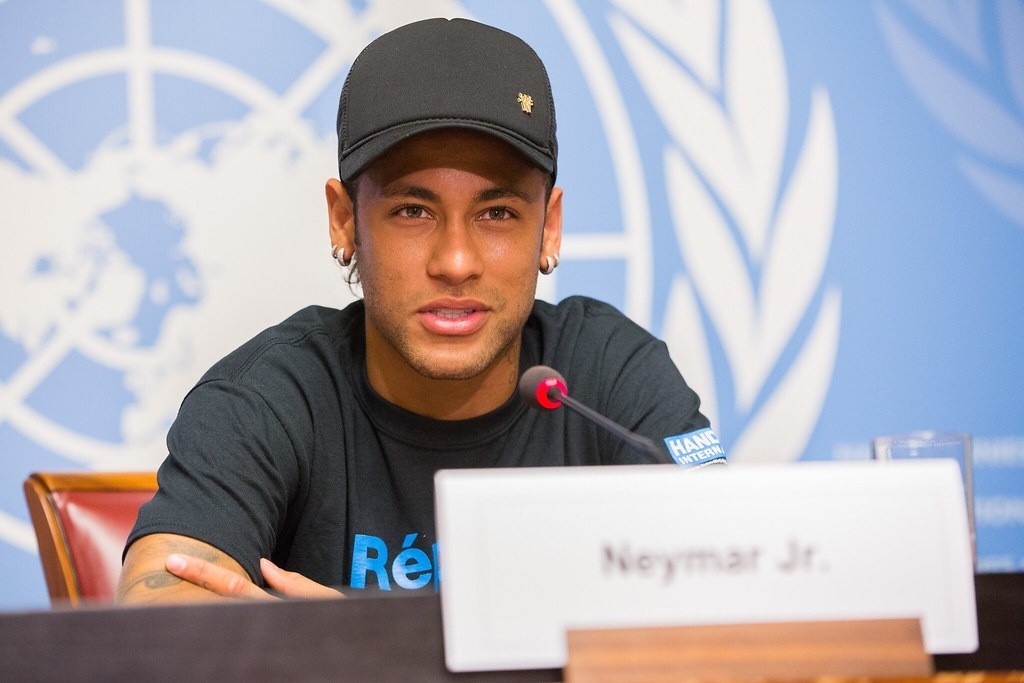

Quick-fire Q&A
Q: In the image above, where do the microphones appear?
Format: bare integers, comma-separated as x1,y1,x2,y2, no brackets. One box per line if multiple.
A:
519,365,672,463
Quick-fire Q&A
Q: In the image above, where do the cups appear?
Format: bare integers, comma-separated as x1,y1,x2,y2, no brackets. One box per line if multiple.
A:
872,429,977,572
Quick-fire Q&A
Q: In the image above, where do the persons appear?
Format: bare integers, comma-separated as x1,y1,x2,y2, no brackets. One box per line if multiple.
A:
116,18,727,609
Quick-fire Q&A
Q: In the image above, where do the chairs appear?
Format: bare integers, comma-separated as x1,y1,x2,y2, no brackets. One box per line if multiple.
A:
21,469,159,607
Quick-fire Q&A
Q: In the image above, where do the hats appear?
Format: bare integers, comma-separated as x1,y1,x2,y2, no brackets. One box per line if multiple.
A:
337,17,557,183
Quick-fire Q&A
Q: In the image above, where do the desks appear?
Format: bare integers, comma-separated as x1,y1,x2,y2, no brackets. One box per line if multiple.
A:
0,574,1024,683
